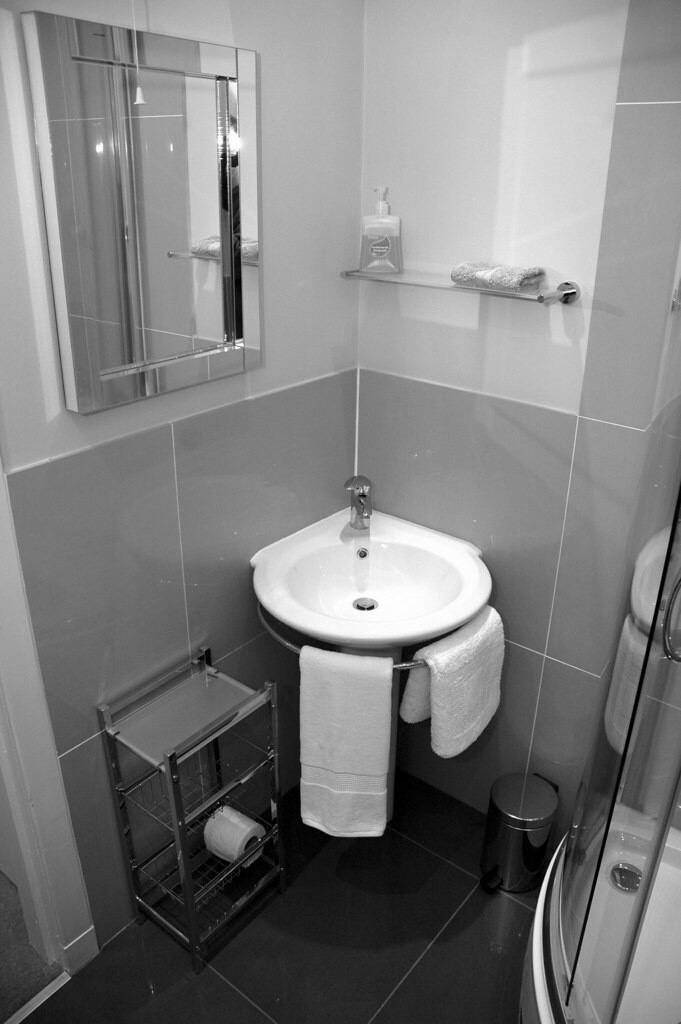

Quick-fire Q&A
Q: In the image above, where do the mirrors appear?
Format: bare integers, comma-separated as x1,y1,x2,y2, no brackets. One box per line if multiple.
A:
20,10,261,413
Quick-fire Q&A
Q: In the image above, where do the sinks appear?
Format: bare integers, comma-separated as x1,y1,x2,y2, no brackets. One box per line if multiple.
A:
532,803,680,1024
630,521,680,647
253,506,493,638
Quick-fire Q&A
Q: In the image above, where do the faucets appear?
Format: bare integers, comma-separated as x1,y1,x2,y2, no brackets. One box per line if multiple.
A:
343,476,379,536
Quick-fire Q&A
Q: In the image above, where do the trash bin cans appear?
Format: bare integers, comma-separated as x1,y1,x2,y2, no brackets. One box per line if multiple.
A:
477,771,559,897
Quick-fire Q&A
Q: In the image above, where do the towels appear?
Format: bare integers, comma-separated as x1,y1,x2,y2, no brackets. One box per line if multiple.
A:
190,232,258,265
297,644,396,839
399,602,504,760
604,616,662,765
449,260,546,289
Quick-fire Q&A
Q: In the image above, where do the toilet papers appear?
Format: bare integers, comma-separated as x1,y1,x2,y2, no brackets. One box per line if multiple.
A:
204,803,267,870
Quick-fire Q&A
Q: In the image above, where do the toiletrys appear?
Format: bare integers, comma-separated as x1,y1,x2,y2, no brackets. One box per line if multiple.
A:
360,185,403,273
